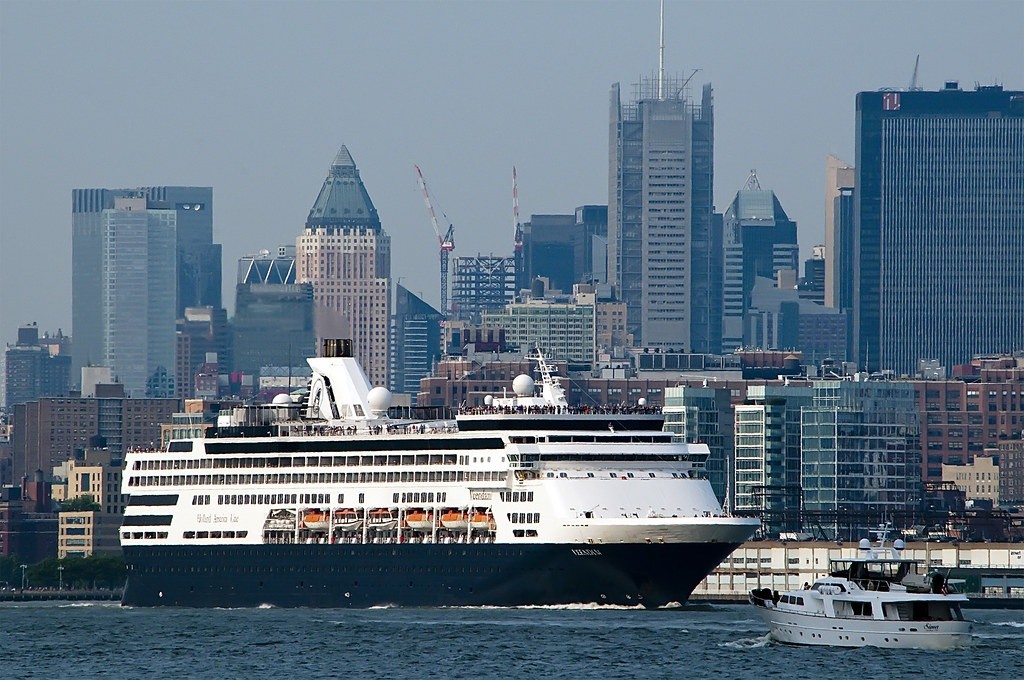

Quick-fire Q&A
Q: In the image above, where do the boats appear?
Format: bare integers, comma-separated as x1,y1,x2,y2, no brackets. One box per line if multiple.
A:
472,515,495,530
441,513,469,530
405,513,435,530
336,511,363,531
369,511,398,531
749,523,975,650
303,514,330,528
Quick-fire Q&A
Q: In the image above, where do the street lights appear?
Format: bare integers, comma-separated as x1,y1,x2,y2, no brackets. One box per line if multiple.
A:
58,564,64,588
20,564,28,590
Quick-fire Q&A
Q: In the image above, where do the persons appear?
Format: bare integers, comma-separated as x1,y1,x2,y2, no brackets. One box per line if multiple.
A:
294,424,458,437
459,401,662,415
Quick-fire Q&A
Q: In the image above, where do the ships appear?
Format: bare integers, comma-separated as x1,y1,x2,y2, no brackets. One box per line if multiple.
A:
117,337,763,610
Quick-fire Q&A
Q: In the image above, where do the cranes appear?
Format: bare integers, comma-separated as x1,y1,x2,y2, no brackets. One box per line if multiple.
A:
508,166,524,299
413,162,456,324
